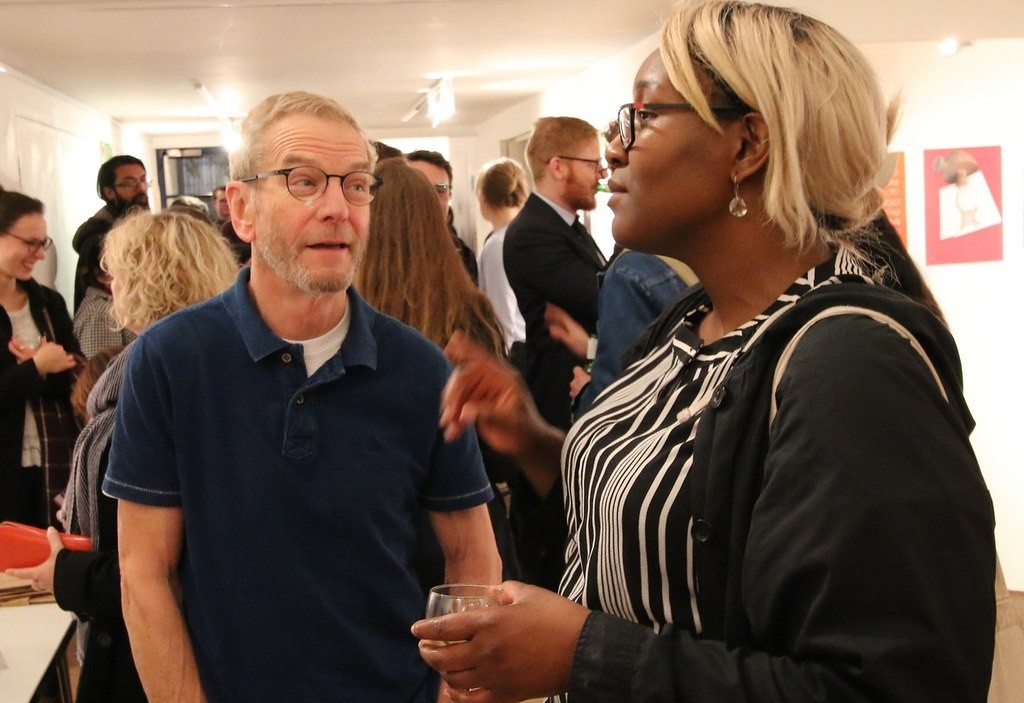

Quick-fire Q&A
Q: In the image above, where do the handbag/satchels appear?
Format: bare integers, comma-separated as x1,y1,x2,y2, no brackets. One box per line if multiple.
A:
766,306,1024,703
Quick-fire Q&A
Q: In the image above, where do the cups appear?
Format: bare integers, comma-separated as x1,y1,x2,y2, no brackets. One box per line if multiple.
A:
425,582,510,692
13,333,42,364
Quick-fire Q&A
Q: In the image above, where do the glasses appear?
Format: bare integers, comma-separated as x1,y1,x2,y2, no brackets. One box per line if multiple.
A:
433,184,451,202
114,178,153,189
618,103,753,152
4,229,53,252
546,155,600,174
239,166,384,206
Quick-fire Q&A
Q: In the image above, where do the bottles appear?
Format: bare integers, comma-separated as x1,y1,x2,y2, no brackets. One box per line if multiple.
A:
584,333,599,373
596,183,610,192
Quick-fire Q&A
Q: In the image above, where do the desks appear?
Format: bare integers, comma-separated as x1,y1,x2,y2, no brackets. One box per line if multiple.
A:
0,594,80,703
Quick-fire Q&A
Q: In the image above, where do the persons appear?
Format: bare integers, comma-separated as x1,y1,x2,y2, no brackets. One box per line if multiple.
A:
0,0,999,703
